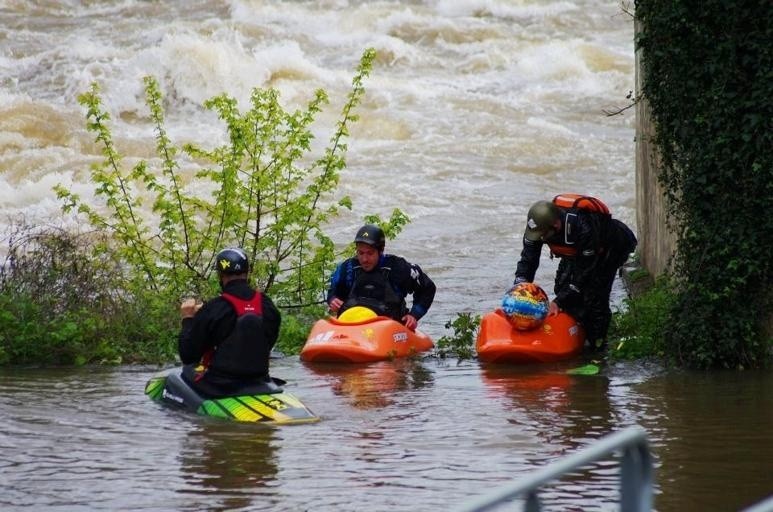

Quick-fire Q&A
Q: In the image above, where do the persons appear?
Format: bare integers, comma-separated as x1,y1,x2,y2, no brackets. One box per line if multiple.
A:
514,190,637,375
177,246,281,396
325,223,436,337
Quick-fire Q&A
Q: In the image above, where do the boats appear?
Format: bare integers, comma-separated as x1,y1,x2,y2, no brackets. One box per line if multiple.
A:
144,362,322,426
300,305,435,366
476,281,585,363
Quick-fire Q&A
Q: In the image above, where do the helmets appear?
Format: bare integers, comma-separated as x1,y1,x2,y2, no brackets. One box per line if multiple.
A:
354,225,385,249
525,200,559,241
215,246,249,274
